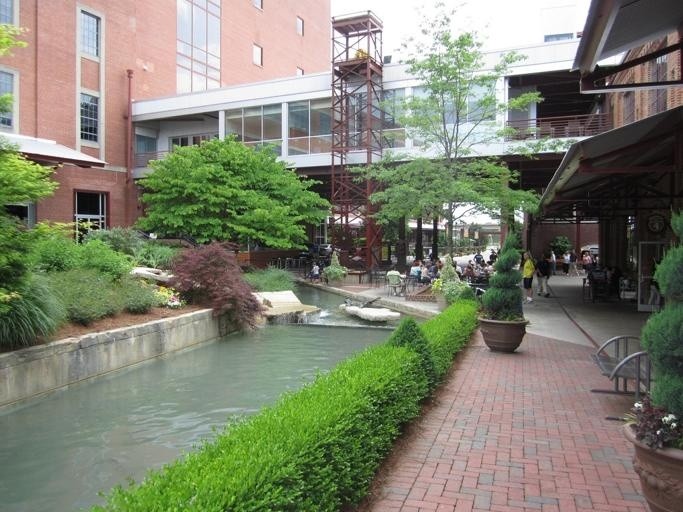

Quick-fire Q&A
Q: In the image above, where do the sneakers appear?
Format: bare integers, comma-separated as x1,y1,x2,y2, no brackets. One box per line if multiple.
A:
524,299,534,304
393,292,403,297
537,292,551,297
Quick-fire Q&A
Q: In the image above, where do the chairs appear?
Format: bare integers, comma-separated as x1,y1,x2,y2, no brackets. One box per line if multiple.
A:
386,274,407,298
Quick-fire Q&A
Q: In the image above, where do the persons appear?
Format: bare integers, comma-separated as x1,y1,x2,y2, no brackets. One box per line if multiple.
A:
308,261,320,283
383,247,599,305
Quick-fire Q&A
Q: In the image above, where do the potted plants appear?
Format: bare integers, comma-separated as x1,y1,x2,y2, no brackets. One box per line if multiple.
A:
476,225,529,352
622,207,682,512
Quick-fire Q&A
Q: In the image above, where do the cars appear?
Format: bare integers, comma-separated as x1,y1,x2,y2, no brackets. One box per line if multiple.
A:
319,244,341,256
